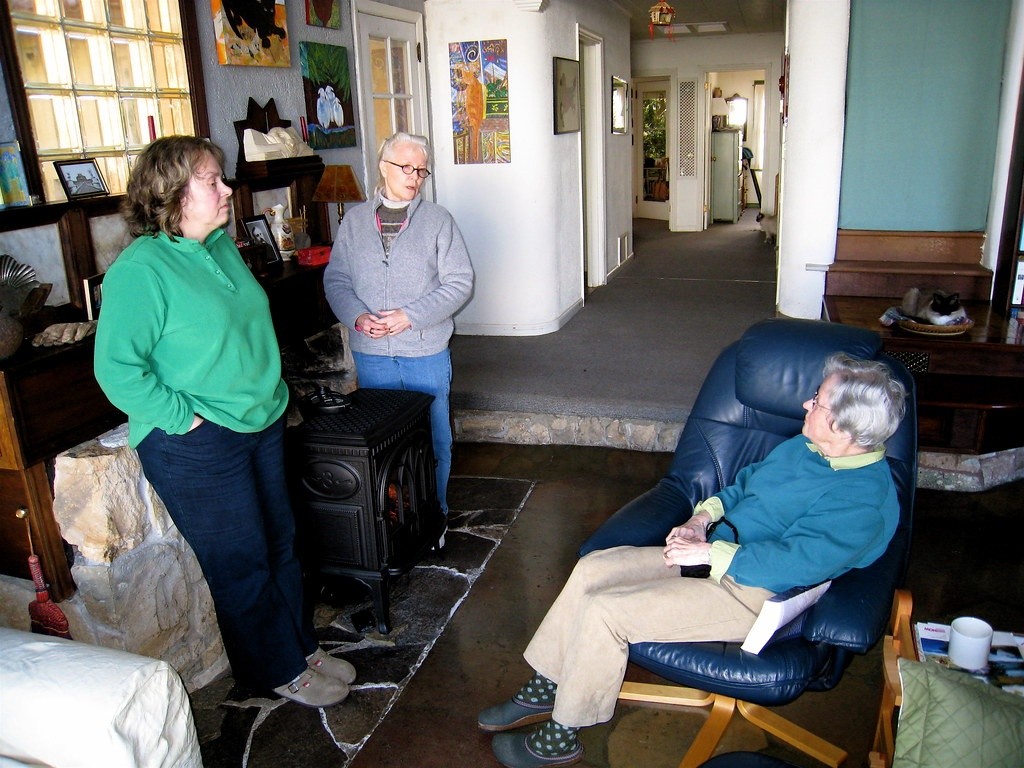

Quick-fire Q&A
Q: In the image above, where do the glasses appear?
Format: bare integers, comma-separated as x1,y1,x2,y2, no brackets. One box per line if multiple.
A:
383,160,432,178
812,384,832,413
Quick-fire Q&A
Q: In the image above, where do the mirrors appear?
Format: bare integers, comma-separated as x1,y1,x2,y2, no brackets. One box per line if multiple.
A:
723,94,748,141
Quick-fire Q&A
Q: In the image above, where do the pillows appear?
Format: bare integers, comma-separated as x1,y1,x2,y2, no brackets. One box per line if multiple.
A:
891,657,1024,768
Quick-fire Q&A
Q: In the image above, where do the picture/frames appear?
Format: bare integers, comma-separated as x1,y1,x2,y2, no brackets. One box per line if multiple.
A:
53,158,111,203
240,213,285,269
83,273,106,320
611,75,628,135
552,56,581,135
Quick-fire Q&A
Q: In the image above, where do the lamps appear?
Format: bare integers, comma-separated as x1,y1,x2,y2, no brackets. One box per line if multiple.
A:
647,0,677,41
312,165,368,225
712,98,731,132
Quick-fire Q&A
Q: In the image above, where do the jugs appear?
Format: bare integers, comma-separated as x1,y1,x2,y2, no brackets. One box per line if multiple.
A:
263,203,296,262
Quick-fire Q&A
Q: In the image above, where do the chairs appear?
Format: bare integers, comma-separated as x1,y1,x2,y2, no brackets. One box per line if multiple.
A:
577,317,919,768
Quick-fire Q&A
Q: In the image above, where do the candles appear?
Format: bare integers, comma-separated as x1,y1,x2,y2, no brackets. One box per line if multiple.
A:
148,116,156,141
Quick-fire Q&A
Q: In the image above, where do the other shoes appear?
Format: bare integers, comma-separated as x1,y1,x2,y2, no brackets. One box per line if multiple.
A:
479,695,555,731
432,525,449,549
490,726,585,768
306,647,356,684
273,669,350,707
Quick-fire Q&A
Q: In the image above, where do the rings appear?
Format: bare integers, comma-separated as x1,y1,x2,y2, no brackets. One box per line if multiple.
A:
371,328,374,334
664,553,669,559
389,328,393,333
371,335,372,338
672,536,676,537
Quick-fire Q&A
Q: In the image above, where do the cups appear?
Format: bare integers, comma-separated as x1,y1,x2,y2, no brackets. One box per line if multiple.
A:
947,616,994,671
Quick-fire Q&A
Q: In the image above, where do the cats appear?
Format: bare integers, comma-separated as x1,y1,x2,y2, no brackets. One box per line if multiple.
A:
902,288,966,325
221,0,287,49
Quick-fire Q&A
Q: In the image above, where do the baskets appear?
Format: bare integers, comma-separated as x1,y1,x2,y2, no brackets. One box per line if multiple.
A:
899,317,975,335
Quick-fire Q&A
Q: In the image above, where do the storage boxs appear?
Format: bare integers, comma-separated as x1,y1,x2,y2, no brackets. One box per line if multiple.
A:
297,246,331,265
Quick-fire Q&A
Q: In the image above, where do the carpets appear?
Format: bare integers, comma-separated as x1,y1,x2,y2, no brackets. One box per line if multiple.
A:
181,475,537,768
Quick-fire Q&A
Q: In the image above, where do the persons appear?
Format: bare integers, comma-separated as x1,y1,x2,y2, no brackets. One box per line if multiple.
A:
473,356,905,767
324,132,474,519
91,137,357,704
252,226,277,263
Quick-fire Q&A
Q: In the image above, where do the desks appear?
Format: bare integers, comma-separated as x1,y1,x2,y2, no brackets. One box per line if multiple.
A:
822,294,1024,449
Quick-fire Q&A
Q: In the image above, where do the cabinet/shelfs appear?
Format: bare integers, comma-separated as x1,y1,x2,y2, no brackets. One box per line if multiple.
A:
712,128,743,224
868,589,1024,768
